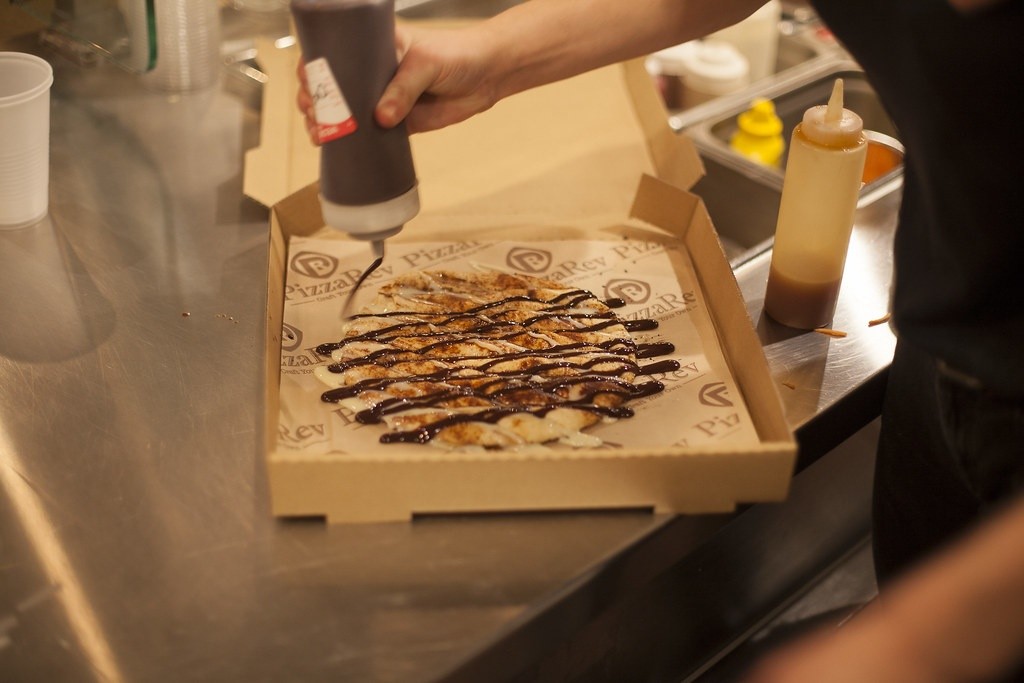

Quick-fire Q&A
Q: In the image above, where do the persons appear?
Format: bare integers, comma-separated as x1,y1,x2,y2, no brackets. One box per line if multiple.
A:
294,0,1024,683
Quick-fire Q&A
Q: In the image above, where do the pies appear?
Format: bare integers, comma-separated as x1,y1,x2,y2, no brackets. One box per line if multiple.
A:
323,265,636,444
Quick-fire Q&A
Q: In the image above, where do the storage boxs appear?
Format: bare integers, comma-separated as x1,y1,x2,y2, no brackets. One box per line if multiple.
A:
240,9,796,524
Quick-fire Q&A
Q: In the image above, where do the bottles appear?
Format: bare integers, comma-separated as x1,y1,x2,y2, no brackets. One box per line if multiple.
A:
762,80,865,329
287,0,419,261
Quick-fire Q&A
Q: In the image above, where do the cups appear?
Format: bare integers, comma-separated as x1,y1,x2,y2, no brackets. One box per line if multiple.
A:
119,0,221,90
0,52,53,231
